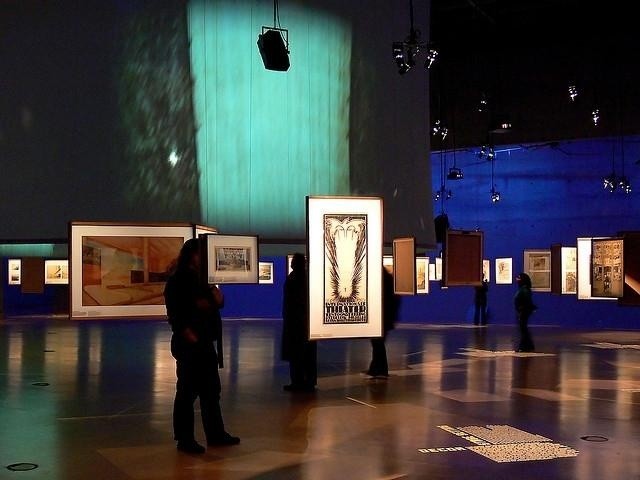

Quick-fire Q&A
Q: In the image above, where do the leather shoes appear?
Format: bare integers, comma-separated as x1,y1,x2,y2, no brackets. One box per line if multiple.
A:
207,431,241,447
282,383,294,392
175,437,207,454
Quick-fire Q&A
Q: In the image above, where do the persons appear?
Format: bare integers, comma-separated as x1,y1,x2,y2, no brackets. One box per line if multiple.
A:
475,273,490,327
361,267,399,377
165,237,239,452
281,253,316,391
514,274,535,353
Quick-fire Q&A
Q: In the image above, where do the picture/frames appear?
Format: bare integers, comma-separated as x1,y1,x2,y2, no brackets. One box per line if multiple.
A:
385,229,513,297
304,194,385,342
206,235,273,286
193,223,218,238
523,236,624,301
7,256,69,294
65,219,193,325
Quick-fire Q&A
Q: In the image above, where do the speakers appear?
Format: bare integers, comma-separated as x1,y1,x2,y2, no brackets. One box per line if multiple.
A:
256,31,291,71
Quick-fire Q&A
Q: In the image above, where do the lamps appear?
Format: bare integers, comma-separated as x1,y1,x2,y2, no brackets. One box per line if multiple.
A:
566,84,603,130
602,142,632,198
476,64,502,206
392,1,439,76
251,0,295,72
433,97,463,204
